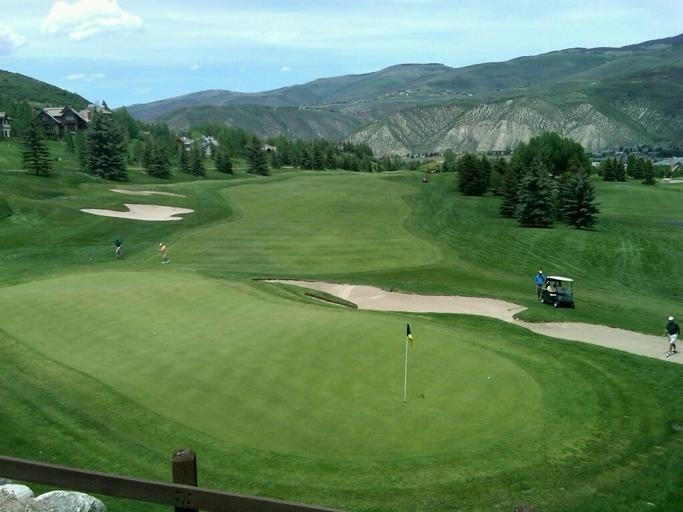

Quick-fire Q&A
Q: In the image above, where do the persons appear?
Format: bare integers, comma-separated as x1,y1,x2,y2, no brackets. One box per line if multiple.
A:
534,269,547,300
159,242,171,264
663,315,681,358
114,238,122,257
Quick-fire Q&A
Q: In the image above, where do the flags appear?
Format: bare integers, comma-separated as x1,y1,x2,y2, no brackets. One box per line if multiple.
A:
407,323,414,346
